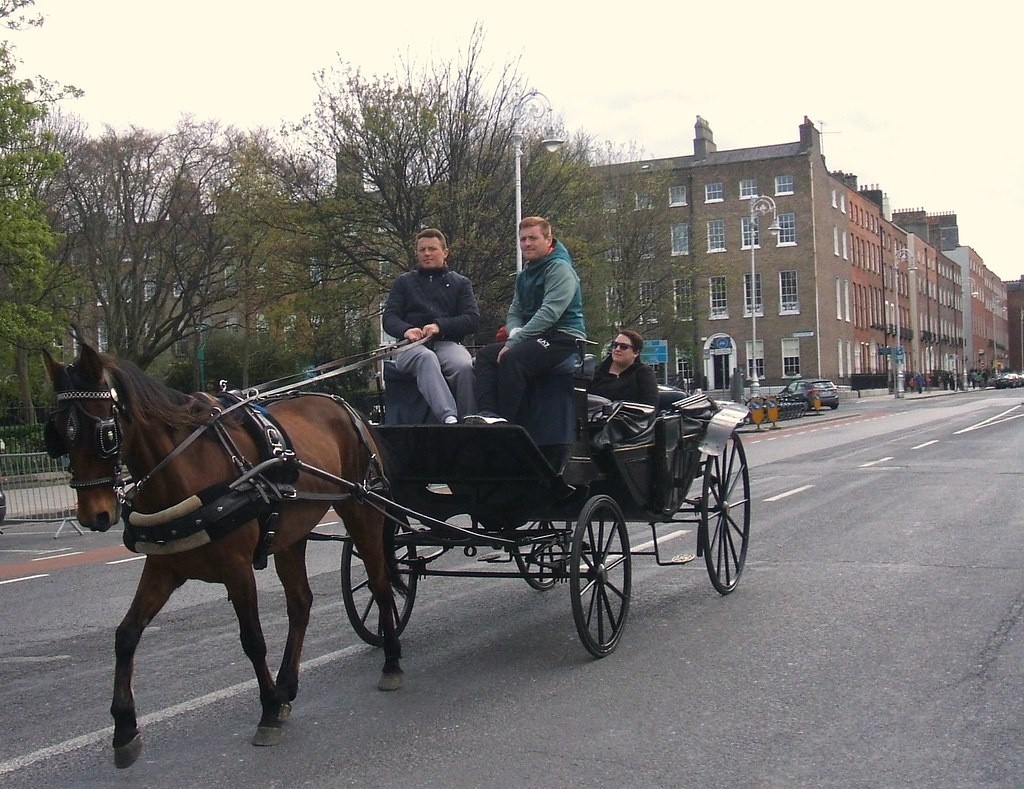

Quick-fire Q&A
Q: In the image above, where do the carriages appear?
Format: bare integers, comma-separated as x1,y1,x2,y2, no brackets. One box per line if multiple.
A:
40,318,751,769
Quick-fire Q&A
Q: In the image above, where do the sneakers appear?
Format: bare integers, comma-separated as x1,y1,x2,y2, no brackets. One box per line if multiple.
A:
463,412,508,424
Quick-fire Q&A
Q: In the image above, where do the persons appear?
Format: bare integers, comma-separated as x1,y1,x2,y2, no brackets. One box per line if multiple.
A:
982,371,988,388
970,369,978,389
460,216,588,424
909,372,925,394
382,230,482,423
591,330,658,451
942,370,963,390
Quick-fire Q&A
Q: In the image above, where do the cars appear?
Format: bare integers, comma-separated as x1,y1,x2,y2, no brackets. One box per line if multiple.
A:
778,379,839,410
993,373,1024,390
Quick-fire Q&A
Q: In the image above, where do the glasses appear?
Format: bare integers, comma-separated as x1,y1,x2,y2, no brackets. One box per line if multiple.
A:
613,340,635,351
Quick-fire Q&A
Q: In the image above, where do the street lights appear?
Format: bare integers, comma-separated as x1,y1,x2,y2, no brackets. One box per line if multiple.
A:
961,277,979,391
991,295,1008,377
196,315,237,392
893,250,919,397
749,195,783,397
509,90,566,277
351,212,384,382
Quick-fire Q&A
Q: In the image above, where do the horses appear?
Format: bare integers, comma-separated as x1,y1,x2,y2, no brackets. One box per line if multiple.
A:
41,341,413,770
